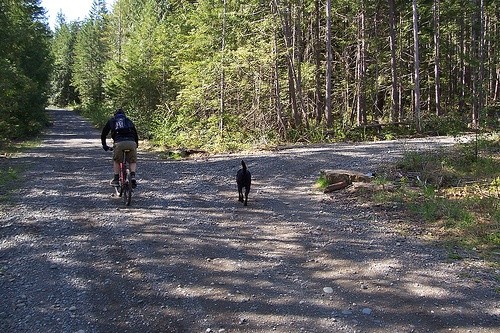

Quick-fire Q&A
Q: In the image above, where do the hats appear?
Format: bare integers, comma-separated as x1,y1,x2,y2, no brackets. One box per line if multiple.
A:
114,109,125,115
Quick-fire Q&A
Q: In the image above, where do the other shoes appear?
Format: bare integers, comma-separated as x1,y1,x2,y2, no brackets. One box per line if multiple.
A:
111,180,119,186
131,177,136,188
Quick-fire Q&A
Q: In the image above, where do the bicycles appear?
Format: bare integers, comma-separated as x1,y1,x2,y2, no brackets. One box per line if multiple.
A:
107,146,137,207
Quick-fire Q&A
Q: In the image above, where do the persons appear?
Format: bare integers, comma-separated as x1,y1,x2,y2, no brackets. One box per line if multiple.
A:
100,109,139,189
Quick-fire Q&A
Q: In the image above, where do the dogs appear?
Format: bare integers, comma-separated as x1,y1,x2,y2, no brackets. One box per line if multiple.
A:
236,160,252,207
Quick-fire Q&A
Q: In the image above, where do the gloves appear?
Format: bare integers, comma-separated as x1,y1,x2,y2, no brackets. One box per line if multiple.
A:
102,144,109,150
